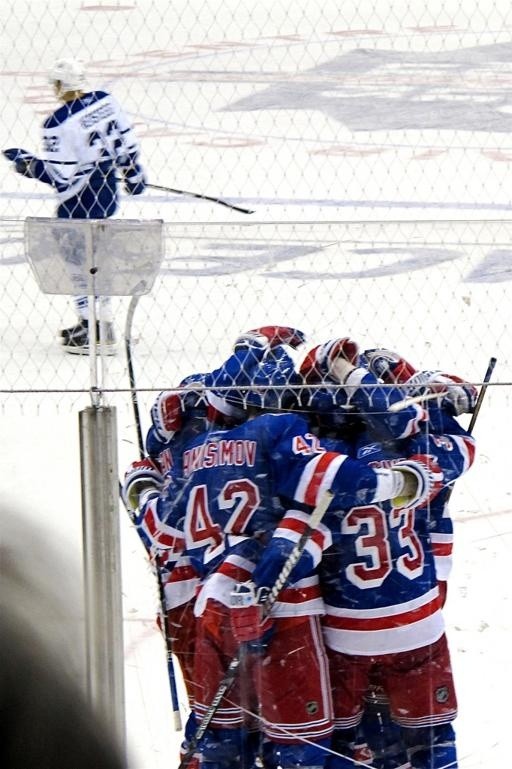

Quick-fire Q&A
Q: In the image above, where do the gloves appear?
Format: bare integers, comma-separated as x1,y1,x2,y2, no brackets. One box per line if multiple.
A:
299,339,478,415
229,578,276,659
389,453,444,510
233,327,304,358
2,147,46,179
151,392,183,443
120,163,146,196
120,456,166,518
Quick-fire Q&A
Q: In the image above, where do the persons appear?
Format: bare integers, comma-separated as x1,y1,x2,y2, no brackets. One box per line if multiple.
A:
0,58,147,356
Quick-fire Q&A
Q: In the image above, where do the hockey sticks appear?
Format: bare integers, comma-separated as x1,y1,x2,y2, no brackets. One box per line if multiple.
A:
115,178,256,214
124,283,182,732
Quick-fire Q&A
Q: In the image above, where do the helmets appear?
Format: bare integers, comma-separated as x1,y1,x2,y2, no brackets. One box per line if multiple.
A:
48,59,83,94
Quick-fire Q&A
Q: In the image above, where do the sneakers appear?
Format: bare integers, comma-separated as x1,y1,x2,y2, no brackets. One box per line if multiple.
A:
56,317,116,349
